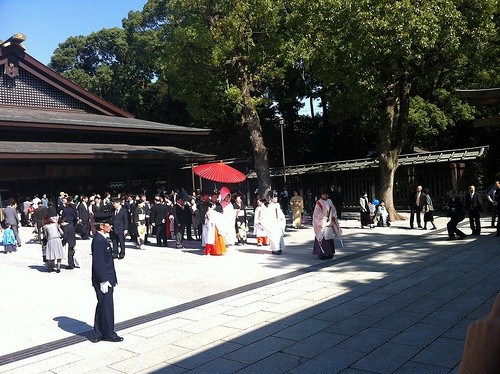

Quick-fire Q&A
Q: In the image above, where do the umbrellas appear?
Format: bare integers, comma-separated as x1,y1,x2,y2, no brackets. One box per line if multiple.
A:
193,160,246,194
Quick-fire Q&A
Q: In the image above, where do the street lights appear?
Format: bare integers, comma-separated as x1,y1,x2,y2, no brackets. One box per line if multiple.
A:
278,116,287,190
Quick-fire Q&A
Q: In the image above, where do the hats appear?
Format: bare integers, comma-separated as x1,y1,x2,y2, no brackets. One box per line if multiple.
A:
95,214,114,226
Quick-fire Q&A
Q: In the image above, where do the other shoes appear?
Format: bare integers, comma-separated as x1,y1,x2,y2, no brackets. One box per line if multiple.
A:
272,250,282,256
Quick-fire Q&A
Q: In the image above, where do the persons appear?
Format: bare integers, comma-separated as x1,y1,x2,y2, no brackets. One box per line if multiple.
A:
359,191,391,229
305,183,342,225
252,188,304,255
407,185,437,230
312,188,344,260
3,186,248,272
444,188,468,240
92,213,124,343
465,186,483,236
456,294,500,374
486,181,500,236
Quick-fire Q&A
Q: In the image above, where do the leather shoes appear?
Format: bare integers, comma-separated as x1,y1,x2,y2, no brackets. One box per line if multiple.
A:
103,335,124,342
94,335,103,343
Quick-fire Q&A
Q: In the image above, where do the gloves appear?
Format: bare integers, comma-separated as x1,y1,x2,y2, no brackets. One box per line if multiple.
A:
100,281,112,294
